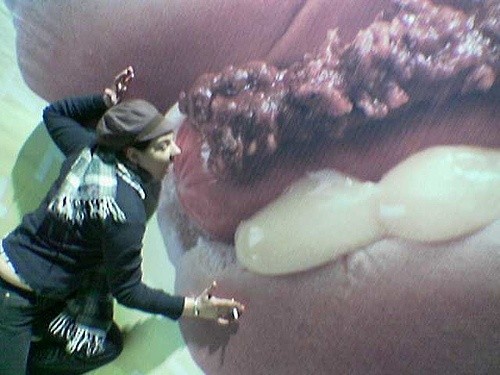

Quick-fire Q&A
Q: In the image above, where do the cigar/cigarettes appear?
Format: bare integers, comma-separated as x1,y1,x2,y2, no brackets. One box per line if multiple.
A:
231,297,238,320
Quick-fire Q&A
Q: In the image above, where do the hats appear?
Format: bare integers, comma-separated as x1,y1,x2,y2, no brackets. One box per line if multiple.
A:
96,98,175,152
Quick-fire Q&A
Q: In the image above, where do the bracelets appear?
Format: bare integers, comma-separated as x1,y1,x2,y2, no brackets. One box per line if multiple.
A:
194,295,200,320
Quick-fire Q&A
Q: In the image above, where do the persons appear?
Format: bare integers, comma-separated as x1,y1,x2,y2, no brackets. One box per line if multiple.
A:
0,66,245,375
